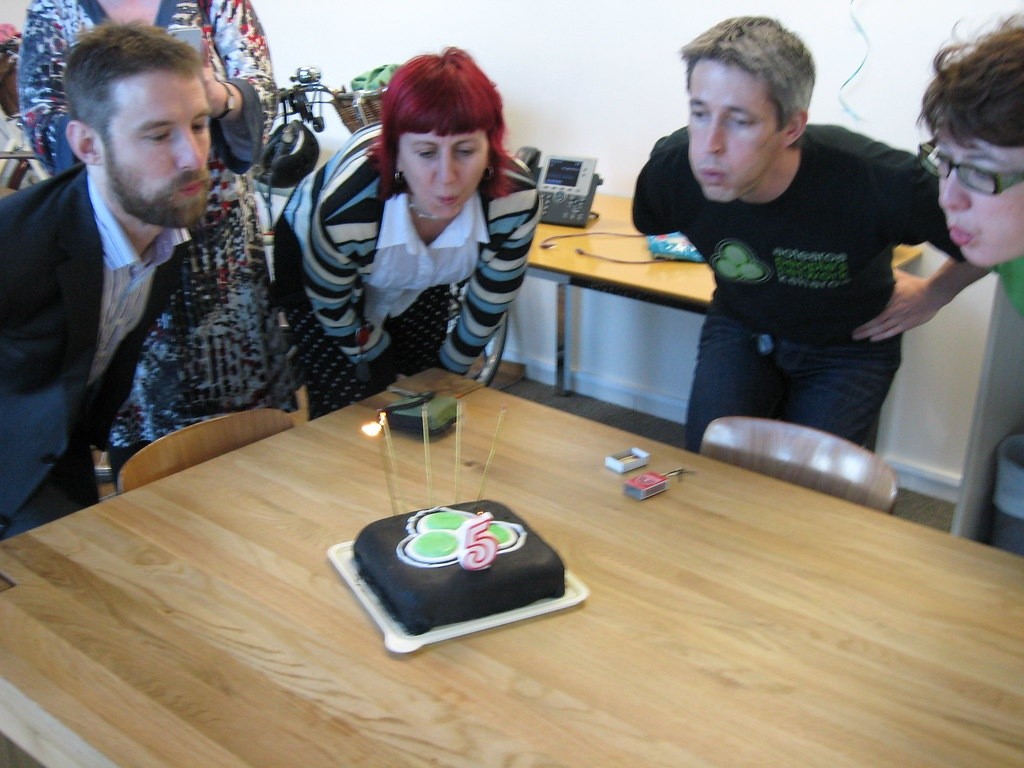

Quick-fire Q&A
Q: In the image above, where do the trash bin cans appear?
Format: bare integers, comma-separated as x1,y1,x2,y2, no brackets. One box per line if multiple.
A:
990,433,1024,556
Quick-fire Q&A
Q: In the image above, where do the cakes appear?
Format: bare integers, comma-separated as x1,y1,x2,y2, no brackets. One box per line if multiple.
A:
352,498,567,634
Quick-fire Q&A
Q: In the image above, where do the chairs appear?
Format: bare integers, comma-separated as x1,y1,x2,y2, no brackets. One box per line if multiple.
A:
115,405,292,496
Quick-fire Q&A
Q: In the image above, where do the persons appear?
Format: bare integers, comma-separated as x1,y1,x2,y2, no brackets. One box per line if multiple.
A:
913,11,1024,320
274,47,546,422
0,22,214,539
630,16,994,459
15,1,303,498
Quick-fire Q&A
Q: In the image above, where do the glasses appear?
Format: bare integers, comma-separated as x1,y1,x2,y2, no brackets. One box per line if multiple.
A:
916,130,1024,197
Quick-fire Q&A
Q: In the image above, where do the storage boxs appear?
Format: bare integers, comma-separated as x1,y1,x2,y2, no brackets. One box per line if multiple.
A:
328,64,400,137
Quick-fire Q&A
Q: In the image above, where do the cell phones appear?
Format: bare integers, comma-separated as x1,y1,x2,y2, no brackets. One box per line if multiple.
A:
166,27,203,56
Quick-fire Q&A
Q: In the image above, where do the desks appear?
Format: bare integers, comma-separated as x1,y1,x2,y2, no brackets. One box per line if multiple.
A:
522,176,717,401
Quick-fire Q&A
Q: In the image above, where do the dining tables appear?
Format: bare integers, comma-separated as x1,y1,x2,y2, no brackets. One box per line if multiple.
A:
0,366,1024,768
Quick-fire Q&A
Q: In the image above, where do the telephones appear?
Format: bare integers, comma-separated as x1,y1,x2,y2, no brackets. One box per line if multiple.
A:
513,146,603,229
